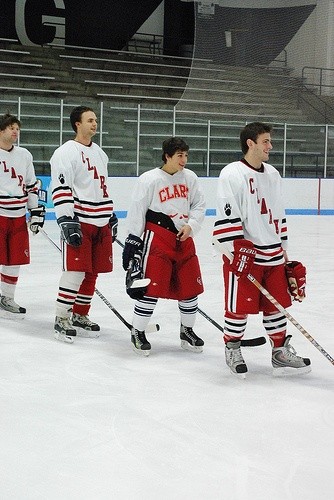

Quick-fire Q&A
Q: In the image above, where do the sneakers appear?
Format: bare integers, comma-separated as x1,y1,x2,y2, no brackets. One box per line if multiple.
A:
225,341,248,381
0,293,26,320
54,316,76,344
72,313,100,338
180,322,204,353
269,334,312,377
130,328,151,357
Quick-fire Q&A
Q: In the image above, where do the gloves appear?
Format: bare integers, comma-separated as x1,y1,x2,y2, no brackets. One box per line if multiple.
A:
109,213,118,244
31,204,46,234
284,260,306,302
57,216,83,248
123,234,151,299
229,239,256,280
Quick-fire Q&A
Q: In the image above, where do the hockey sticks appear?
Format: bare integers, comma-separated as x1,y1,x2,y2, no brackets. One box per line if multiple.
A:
211,237,334,366
114,237,266,347
25,221,162,335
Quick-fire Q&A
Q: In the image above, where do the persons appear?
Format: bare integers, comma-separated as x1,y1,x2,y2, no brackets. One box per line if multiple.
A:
122,138,207,357
0,114,46,319
212,122,312,380
50,106,119,345
